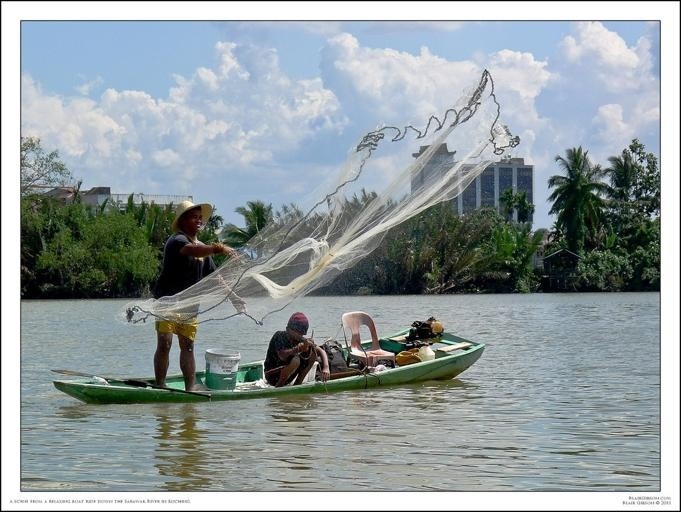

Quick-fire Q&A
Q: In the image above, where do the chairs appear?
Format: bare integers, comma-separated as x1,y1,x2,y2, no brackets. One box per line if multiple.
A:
341,311,396,373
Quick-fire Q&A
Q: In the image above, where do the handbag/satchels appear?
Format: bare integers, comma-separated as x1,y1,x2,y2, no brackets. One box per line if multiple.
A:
317,340,348,371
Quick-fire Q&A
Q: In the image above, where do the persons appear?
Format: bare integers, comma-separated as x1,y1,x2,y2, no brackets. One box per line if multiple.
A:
262,313,331,389
152,201,250,388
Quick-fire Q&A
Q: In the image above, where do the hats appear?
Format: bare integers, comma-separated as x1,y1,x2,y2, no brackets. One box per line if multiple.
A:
172,201,212,229
288,312,308,335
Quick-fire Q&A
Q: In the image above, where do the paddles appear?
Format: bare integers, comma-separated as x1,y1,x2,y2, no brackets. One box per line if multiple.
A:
51,368,212,398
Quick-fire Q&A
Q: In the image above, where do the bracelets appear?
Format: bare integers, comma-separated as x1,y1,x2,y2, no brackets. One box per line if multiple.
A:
213,240,229,256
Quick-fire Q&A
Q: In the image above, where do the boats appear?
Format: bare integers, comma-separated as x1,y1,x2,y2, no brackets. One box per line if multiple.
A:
50,323,488,404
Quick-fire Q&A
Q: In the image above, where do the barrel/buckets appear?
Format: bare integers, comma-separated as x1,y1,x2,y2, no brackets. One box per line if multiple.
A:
204,348,242,391
417,343,435,362
